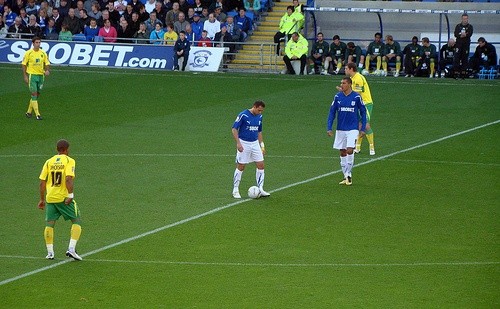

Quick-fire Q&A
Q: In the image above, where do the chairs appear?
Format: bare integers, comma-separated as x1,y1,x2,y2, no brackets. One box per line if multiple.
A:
361,49,497,80
227,11,254,20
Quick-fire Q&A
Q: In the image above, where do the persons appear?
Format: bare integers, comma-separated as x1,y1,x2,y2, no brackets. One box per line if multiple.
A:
454,13,474,80
326,76,367,186
21,37,49,120
231,99,271,199
468,37,497,78
173,31,190,72
0,0,271,73
335,62,375,156
38,139,83,261
273,0,456,79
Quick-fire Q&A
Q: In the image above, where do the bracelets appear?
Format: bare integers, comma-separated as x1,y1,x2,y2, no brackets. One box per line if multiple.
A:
260,143,265,147
68,193,74,198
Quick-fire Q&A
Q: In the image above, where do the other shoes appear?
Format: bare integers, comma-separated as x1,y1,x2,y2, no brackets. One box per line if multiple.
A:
354,149,361,154
36,114,43,120
370,149,375,156
338,176,352,186
25,113,32,118
285,68,435,78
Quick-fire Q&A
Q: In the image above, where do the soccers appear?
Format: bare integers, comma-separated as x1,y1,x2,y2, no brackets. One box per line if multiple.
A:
247,186,261,199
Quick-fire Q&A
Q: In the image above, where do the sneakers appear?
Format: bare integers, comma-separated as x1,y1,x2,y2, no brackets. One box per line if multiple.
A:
231,190,242,199
45,252,55,260
65,249,83,261
260,189,270,197
